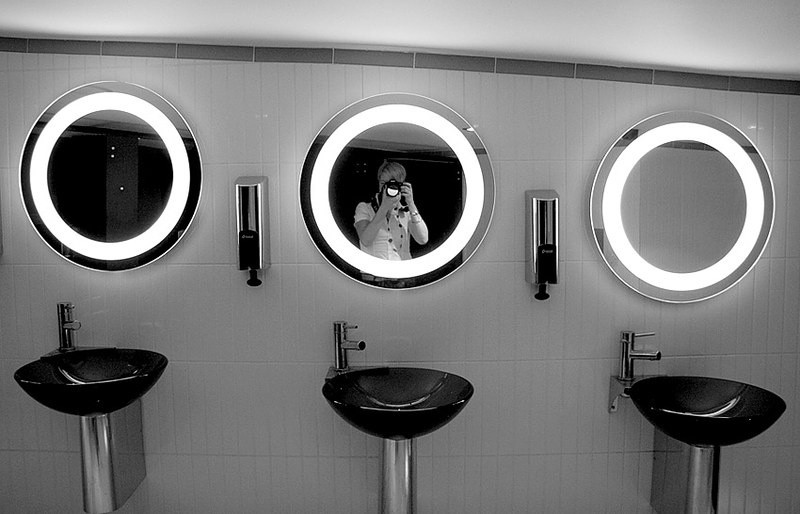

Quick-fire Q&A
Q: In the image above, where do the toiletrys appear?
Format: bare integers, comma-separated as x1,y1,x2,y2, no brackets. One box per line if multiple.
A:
524,189,560,301
235,175,271,287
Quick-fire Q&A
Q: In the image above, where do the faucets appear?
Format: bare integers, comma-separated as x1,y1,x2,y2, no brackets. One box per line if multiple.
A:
617,330,662,381
332,320,366,372
56,301,82,352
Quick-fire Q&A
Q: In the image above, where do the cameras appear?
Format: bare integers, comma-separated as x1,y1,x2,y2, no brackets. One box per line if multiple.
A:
385,178,403,197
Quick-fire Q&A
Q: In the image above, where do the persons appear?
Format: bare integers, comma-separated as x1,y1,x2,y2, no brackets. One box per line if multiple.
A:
353,162,429,289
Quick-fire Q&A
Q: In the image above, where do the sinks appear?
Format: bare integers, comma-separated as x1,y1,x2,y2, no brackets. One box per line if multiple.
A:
321,367,474,440
14,346,168,416
629,376,787,447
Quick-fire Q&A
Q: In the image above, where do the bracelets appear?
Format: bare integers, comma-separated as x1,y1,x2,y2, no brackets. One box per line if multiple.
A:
411,221,421,223
410,211,419,216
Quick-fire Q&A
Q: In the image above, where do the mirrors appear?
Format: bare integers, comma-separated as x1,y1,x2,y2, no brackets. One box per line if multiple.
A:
18,82,201,273
589,111,776,305
295,94,496,293
